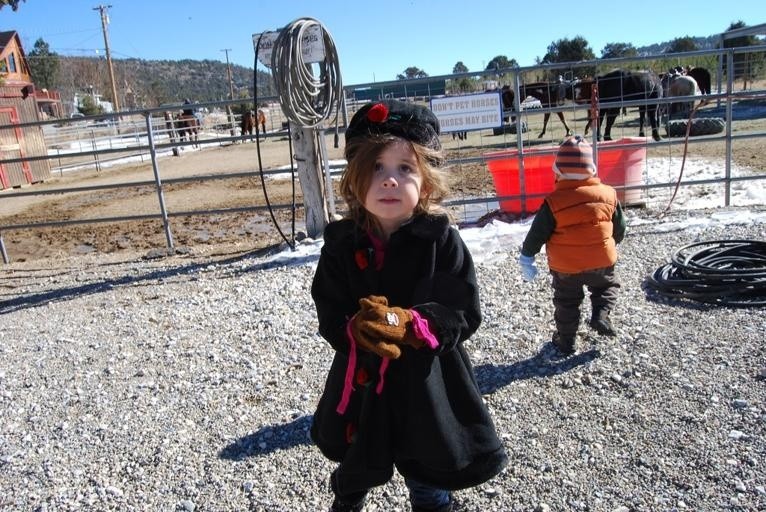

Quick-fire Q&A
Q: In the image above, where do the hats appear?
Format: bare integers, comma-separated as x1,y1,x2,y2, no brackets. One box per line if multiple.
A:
552,135,597,180
345,99,441,167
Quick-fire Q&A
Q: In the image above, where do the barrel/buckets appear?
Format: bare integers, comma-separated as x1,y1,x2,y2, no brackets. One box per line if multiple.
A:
481,147,560,216
588,136,651,206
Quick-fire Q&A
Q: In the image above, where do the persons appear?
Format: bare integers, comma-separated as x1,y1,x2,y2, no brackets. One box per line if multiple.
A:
519,135,626,353
174,112,185,144
181,98,201,129
307,99,509,512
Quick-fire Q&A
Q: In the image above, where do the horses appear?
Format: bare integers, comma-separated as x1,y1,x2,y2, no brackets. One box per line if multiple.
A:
451,66,712,141
174,114,200,151
241,109,266,144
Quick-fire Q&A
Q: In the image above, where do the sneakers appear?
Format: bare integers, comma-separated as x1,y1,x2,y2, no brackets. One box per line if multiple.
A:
590,315,616,337
552,331,577,353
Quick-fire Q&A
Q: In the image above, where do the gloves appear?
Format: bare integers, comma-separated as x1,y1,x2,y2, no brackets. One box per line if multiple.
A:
351,295,427,359
519,251,540,280
